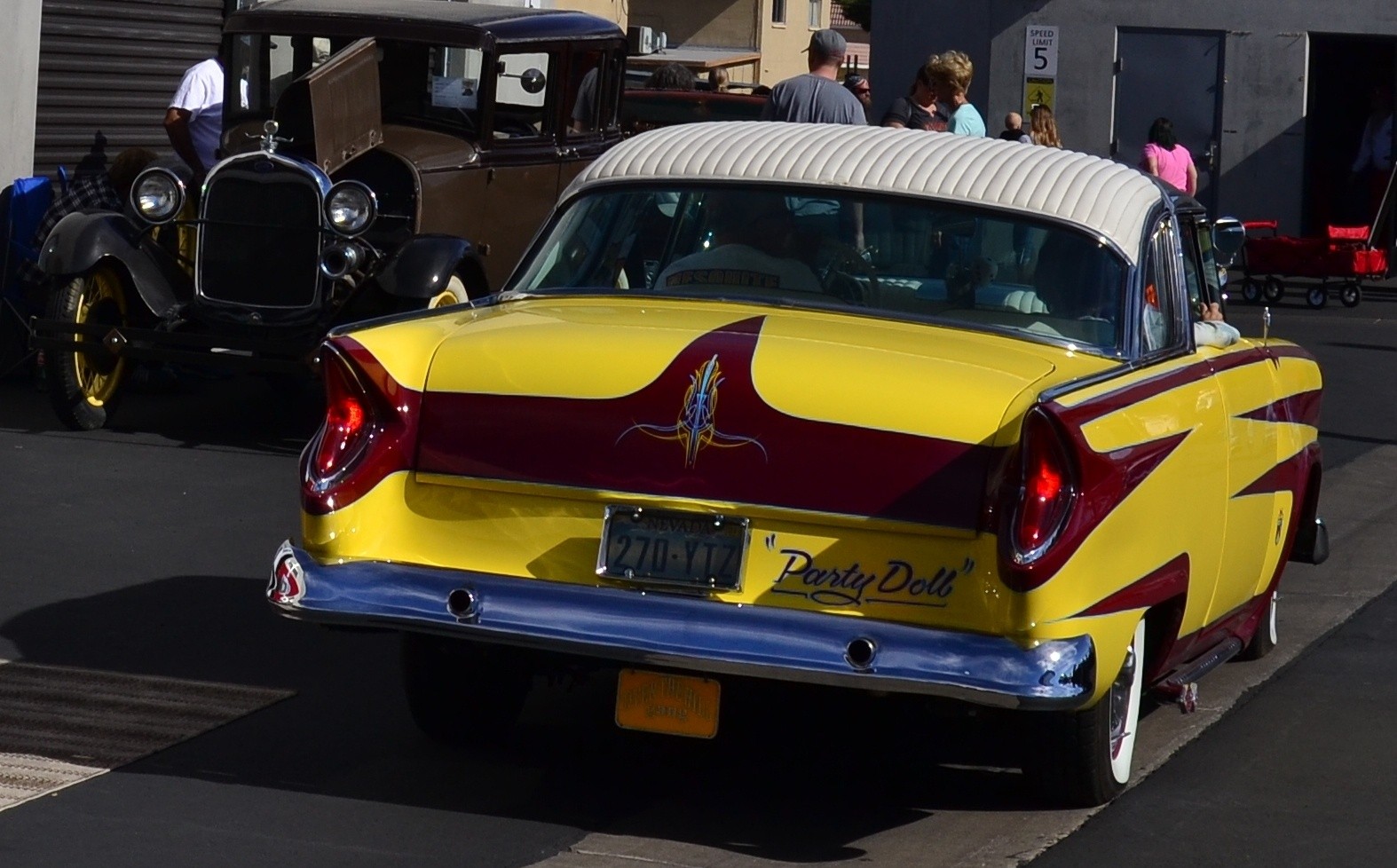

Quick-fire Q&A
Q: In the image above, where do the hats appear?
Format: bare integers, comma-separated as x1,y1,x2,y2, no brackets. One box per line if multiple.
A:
237,32,276,50
801,29,848,57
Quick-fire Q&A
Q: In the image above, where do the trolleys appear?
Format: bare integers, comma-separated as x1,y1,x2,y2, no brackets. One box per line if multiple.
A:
1230,217,1387,309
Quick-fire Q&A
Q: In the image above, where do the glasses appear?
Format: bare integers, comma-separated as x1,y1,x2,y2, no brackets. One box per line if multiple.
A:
854,87,872,94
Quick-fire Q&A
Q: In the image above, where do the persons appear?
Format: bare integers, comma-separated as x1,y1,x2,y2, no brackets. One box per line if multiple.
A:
1012,103,1063,286
163,33,278,190
707,66,731,93
1344,76,1397,263
878,62,953,277
998,111,1032,145
642,60,696,91
1135,116,1198,198
842,72,876,126
461,79,474,96
923,49,987,277
13,147,166,394
1030,225,1242,354
651,186,824,294
756,28,870,269
569,66,599,134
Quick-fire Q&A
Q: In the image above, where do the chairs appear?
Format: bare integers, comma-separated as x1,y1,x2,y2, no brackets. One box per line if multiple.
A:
940,308,1118,345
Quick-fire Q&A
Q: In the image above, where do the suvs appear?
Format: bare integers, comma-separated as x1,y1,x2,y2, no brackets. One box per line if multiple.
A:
24,2,628,437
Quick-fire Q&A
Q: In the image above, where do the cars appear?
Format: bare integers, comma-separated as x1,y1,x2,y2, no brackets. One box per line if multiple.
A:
270,119,1332,804
